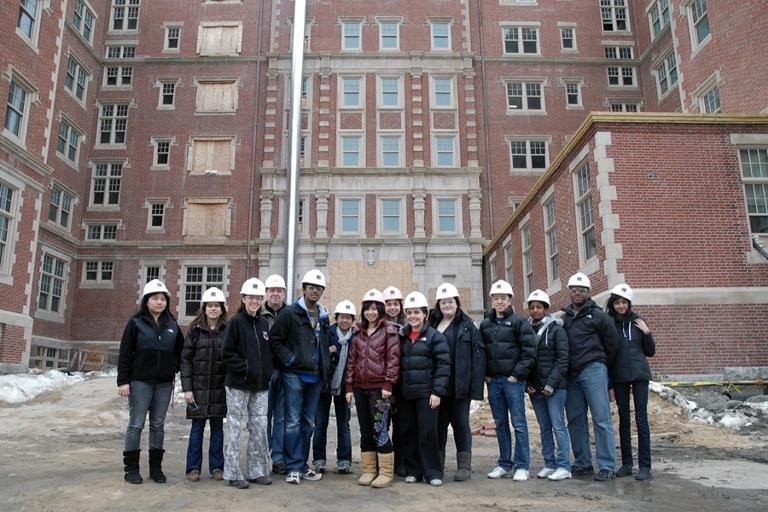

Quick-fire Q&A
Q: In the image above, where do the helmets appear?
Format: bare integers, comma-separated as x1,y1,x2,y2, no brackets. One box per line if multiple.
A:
610,283,634,305
489,279,551,309
301,269,327,291
200,274,286,313
435,283,460,305
140,279,172,303
567,272,592,292
334,285,430,320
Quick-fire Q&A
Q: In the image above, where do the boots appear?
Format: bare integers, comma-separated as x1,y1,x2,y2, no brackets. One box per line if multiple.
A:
149,448,166,483
358,452,471,488
123,449,143,484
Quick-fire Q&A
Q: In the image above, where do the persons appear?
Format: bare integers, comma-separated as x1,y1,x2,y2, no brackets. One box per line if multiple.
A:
558,272,620,481
479,280,537,482
429,282,485,482
525,289,573,480
178,287,230,483
220,267,451,489
605,283,655,481
116,280,183,483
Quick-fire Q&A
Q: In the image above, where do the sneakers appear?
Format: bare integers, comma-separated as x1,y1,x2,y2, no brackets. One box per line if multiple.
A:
229,460,350,488
537,465,651,480
188,470,199,482
487,466,531,481
213,471,222,480
